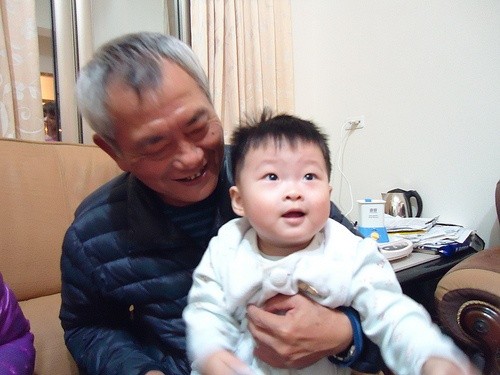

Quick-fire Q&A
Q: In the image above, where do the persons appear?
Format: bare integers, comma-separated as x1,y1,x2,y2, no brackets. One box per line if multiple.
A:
59,32,383,375
0,272,36,375
182,115,470,375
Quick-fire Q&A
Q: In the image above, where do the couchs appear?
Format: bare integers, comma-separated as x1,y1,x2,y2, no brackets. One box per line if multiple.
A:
433,179,500,375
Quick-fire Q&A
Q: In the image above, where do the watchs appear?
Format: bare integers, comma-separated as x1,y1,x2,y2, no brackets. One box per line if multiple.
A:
333,344,357,362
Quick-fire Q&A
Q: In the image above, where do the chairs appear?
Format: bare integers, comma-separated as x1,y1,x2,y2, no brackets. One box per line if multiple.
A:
0,137,125,375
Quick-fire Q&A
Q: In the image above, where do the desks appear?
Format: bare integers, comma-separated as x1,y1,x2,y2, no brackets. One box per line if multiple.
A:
393,241,483,283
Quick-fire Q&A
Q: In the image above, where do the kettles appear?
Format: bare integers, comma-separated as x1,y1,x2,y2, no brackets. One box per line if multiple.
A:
381,188,423,218
